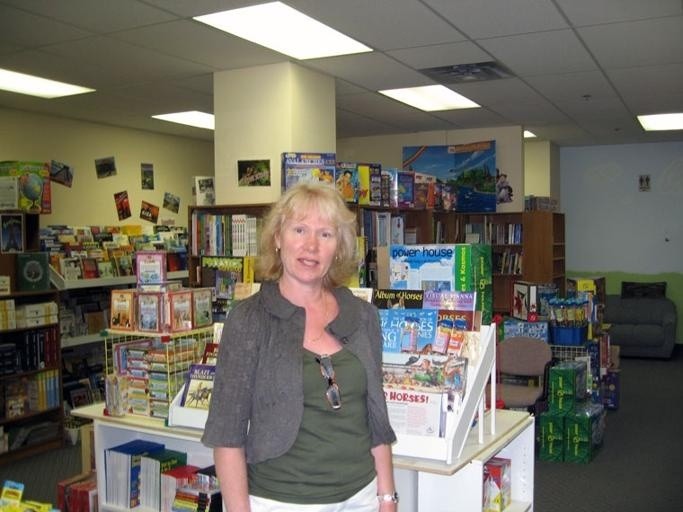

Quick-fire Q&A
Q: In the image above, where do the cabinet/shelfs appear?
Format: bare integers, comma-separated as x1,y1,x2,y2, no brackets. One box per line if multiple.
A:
70,322,536,512
187,204,564,324
1,215,188,465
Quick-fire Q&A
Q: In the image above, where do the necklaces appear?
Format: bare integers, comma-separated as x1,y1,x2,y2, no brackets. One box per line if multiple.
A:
302,290,330,347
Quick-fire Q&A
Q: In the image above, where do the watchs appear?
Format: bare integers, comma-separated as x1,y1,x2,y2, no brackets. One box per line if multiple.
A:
375,490,399,505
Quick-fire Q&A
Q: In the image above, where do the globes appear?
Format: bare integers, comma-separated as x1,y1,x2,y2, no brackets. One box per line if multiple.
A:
19,172,43,214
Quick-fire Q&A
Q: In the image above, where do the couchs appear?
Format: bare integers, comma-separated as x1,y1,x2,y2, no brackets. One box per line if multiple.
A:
604,296,677,358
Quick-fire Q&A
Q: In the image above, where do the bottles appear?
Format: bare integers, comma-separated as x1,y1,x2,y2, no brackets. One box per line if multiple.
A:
399,316,468,355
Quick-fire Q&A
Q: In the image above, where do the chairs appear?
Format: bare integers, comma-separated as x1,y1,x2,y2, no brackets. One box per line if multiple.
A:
485,338,556,413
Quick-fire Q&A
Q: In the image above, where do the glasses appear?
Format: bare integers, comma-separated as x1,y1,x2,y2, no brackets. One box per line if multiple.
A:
315,353,342,410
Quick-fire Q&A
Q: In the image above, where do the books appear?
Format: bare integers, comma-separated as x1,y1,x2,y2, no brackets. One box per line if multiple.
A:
192,213,263,320
2,214,59,329
56,292,113,426
106,250,212,419
101,438,223,512
39,224,188,279
182,324,224,409
2,421,59,452
355,204,524,441
2,327,60,419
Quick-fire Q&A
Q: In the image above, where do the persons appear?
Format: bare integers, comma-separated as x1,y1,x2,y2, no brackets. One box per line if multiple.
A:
335,169,355,200
200,173,401,512
